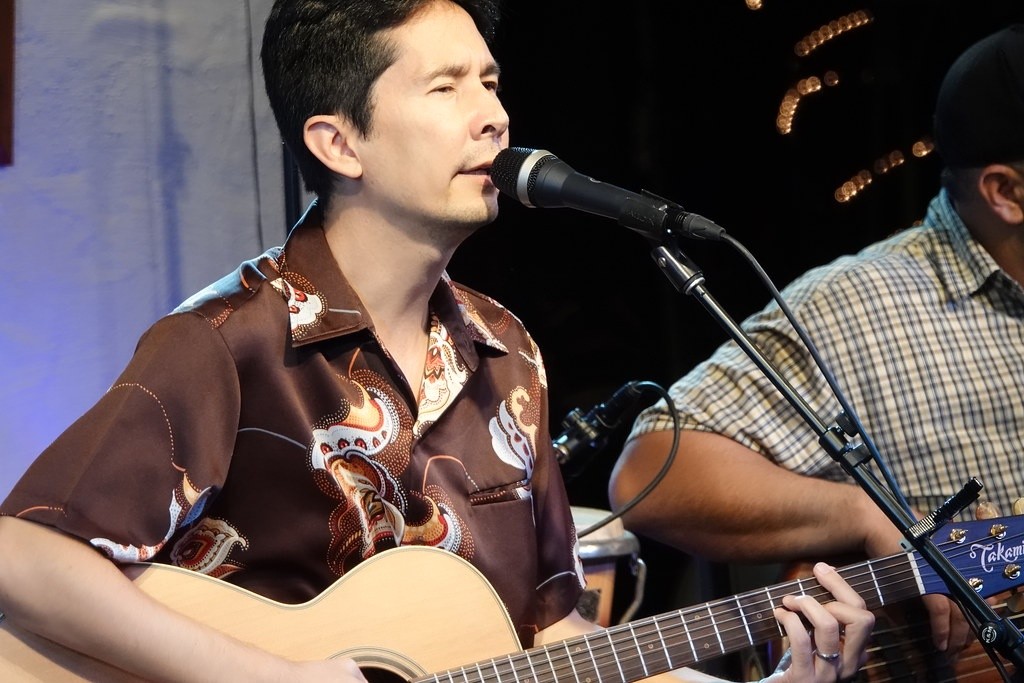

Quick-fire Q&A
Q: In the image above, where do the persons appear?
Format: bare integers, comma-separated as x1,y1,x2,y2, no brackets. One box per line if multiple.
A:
608,84,1024,683
1,1,876,683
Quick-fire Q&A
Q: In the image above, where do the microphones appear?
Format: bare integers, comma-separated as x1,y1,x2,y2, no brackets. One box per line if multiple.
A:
489,147,726,242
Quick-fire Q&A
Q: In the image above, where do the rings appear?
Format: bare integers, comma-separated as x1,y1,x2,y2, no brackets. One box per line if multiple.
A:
813,649,842,663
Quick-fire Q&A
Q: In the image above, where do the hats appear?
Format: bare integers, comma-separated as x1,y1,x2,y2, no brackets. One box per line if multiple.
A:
932,26,1024,169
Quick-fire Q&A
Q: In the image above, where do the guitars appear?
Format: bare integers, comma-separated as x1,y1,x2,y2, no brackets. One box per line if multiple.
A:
0,496,1024,682
